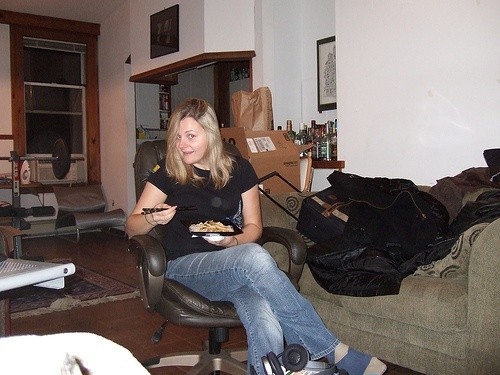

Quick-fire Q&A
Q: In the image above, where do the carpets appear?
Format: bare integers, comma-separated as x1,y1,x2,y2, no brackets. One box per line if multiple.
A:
10,258,141,321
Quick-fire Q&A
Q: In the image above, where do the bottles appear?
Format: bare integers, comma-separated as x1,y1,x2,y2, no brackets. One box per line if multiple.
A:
160,93,169,110
277,119,337,162
230,67,250,81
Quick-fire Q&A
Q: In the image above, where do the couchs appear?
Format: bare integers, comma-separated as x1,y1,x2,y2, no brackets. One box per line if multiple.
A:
260,186,500,375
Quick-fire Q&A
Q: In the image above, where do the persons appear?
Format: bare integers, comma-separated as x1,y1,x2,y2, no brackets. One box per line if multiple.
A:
124,99,387,375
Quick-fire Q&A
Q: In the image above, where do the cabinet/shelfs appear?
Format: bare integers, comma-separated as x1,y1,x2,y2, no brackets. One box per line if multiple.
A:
129,51,256,204
0,180,42,260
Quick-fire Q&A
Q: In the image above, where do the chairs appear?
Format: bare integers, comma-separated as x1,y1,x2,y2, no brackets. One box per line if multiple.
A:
123,141,307,375
53,182,127,245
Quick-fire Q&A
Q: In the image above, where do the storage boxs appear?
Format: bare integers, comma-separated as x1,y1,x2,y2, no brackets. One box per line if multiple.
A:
220,128,316,196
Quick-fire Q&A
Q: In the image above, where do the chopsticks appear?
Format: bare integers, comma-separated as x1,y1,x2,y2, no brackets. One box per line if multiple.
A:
141,205,199,214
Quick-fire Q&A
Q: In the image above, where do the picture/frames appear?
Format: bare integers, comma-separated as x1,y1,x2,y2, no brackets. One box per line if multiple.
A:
317,36,337,112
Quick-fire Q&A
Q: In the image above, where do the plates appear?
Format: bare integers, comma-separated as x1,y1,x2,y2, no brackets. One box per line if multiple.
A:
179,218,244,237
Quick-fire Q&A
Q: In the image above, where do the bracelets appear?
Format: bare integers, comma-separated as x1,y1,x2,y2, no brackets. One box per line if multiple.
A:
145,214,156,227
233,237,238,245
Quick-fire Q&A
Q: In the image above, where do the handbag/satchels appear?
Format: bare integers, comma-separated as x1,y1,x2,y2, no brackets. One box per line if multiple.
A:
296,187,349,244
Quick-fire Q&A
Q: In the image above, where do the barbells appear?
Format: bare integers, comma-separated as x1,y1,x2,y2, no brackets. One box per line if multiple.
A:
0,137,85,180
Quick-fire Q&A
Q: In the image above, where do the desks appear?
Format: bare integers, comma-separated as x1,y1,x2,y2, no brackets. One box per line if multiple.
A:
0,257,76,338
